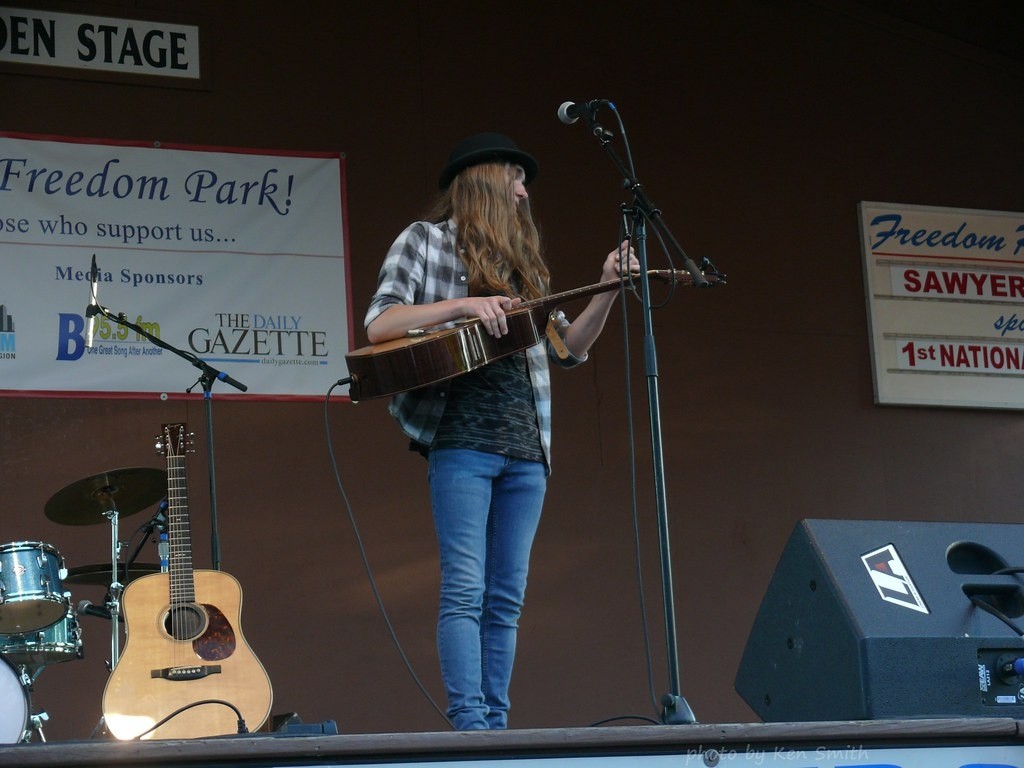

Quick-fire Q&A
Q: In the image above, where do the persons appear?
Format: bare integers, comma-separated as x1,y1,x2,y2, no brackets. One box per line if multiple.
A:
362,123,639,730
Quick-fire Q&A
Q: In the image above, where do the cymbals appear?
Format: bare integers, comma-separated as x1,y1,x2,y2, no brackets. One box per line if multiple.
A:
57,561,165,583
45,465,168,523
82,602,124,621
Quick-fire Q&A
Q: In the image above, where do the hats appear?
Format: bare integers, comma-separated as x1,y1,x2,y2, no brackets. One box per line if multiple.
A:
439,131,540,194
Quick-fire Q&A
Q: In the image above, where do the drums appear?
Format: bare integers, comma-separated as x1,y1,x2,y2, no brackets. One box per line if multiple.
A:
0,654,35,746
0,541,67,633
0,608,84,667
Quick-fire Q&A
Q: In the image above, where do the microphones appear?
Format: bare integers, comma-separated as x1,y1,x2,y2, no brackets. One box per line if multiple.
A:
84,263,99,347
557,99,610,125
76,599,123,622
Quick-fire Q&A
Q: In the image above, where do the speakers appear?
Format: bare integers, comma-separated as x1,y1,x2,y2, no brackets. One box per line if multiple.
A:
733,518,1024,723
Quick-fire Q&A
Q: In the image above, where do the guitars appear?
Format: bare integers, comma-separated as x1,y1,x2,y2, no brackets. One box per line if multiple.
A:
103,423,274,741
346,268,696,401
